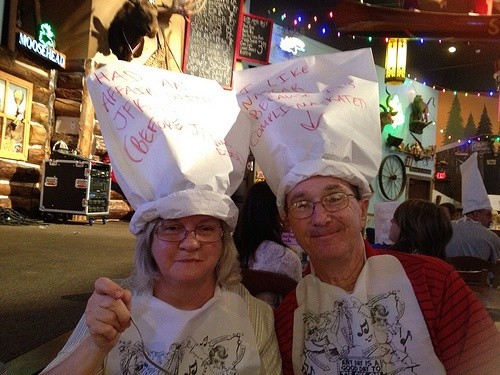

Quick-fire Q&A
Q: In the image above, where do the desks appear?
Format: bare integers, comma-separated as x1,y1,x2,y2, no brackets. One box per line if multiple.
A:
464,283,500,322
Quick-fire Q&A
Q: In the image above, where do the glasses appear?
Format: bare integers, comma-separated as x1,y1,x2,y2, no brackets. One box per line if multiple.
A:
152,222,225,243
390,218,398,223
285,191,360,219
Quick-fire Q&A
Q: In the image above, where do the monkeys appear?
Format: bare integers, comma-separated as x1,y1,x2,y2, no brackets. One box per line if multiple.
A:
410,94,436,123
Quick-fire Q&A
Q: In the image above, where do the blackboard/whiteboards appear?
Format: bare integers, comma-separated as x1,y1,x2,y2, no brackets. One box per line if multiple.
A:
183,1,244,89
240,11,275,67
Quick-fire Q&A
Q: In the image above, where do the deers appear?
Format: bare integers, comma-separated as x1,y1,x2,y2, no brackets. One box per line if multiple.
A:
154,0,207,47
379,84,398,133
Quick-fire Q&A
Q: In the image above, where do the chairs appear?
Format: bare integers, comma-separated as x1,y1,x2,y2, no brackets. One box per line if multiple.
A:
243,272,297,307
449,255,500,287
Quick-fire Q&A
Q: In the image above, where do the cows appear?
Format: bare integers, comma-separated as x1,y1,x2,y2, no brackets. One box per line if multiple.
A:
106,0,159,62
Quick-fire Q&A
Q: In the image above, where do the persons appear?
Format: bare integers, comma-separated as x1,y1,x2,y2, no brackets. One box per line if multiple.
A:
445,153,500,288
440,203,456,221
271,175,500,375
37,215,282,375
232,181,303,310
373,198,453,263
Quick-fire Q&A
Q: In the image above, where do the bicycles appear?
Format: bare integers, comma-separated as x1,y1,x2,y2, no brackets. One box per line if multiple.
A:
0,205,50,226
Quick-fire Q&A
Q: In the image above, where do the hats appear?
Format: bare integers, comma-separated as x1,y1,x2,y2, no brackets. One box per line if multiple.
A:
85,61,251,235
460,151,493,215
231,47,383,220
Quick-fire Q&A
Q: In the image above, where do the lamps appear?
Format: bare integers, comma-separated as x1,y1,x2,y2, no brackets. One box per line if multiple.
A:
384,38,407,86
